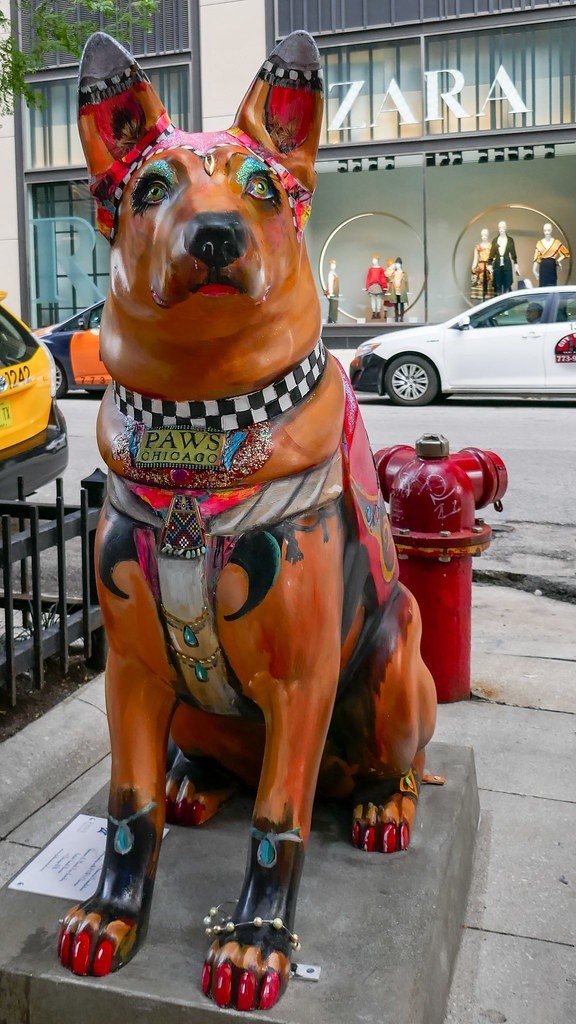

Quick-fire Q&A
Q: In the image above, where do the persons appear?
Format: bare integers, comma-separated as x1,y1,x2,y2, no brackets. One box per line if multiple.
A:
470,229,496,299
487,220,520,297
383,258,399,322
526,303,544,324
327,260,340,324
363,252,388,320
531,223,569,287
390,257,409,322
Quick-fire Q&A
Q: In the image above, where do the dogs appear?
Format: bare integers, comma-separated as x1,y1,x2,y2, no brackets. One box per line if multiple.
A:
54,26,446,1011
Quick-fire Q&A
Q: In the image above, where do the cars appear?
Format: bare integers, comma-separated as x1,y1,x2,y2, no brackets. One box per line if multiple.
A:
348,286,576,407
33,298,112,399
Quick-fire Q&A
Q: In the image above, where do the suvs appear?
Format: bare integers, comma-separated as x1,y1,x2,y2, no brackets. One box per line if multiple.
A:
0,291,68,501
